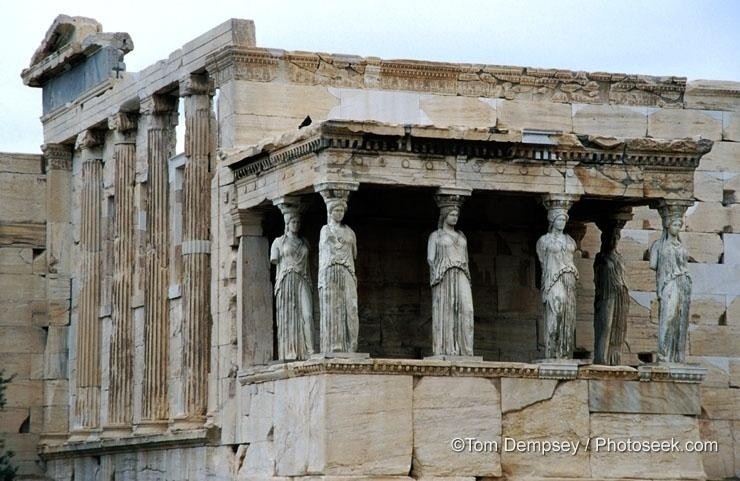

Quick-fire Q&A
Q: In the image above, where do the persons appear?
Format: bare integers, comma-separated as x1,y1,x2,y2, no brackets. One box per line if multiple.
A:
426,206,474,356
317,200,359,354
535,209,579,359
270,212,315,361
593,228,629,366
649,215,693,364
